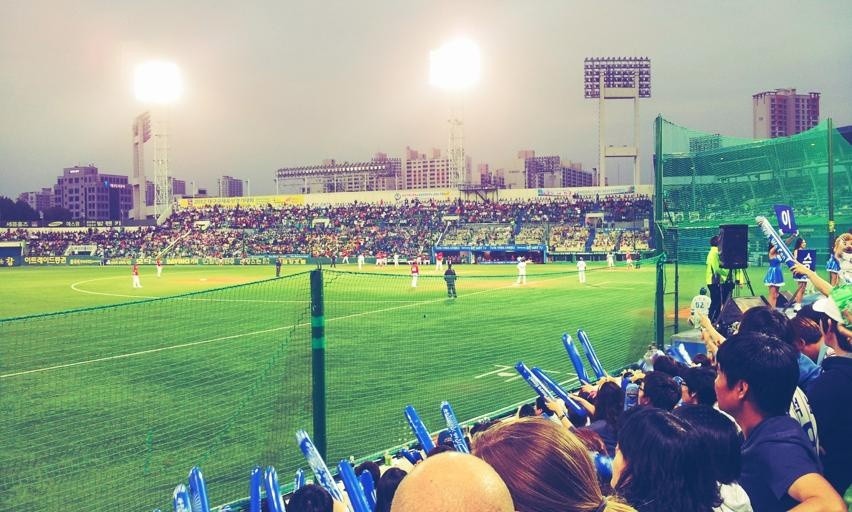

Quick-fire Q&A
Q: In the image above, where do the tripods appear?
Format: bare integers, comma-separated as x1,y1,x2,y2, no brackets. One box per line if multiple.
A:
726,269,754,297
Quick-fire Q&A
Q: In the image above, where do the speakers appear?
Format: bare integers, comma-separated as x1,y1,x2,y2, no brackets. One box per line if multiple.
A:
714,296,771,325
719,224,749,269
776,290,796,307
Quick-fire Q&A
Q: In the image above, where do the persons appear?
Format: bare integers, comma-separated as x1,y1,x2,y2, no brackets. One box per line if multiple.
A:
0,172,852,511
